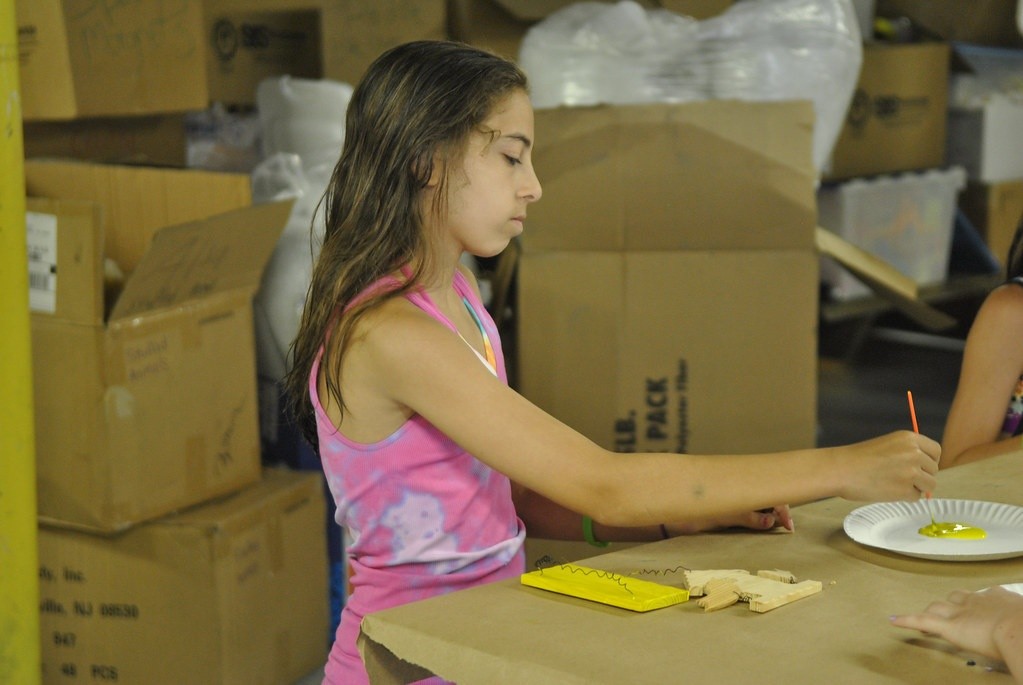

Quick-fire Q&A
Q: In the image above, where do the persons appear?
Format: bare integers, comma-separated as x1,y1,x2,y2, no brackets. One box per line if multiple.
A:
937,206,1023,470
280,39,944,685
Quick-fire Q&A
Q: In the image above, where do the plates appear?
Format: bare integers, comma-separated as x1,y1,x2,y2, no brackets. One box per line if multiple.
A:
843,498,1023,562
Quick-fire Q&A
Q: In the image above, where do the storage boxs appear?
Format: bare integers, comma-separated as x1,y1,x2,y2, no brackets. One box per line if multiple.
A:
14,0,1023,685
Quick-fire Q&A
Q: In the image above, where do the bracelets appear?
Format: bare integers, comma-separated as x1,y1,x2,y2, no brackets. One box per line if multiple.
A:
660,523,669,539
581,514,611,548
